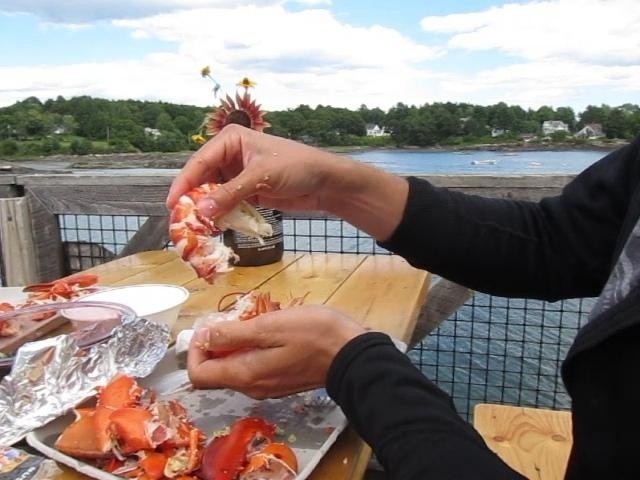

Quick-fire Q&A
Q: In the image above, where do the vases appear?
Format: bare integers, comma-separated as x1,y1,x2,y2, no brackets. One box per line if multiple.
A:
221,203,284,268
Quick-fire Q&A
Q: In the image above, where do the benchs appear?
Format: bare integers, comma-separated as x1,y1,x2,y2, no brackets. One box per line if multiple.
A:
474,404,574,479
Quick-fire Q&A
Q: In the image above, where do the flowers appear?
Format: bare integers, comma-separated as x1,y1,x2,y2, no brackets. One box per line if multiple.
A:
190,65,275,171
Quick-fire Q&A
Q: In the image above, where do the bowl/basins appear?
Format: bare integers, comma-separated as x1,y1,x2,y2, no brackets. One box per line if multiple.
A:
0,301,134,375
65,281,192,338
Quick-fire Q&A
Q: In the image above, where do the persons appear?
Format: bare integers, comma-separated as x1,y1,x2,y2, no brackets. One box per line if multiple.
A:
165,122,640,480
